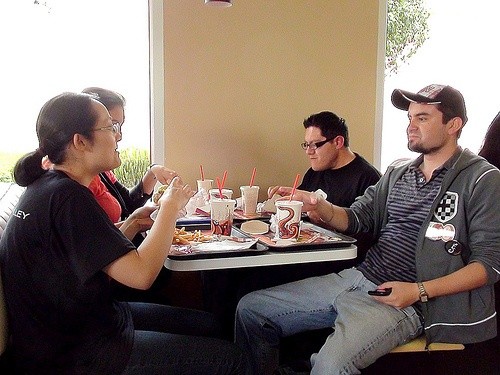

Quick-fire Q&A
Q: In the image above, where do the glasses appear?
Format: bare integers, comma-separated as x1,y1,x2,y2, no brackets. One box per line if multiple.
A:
301,139,333,150
94,122,121,135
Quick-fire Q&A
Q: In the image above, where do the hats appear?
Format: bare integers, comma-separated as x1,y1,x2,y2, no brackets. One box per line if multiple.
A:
391,84,468,128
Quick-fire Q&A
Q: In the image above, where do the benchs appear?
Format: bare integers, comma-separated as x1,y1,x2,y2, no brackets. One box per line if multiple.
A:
0,179,27,242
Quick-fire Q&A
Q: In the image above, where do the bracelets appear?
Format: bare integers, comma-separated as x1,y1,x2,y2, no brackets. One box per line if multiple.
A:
149,163,158,168
319,202,334,223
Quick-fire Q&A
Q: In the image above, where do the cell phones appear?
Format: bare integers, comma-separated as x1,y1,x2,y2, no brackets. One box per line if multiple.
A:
368,288,392,296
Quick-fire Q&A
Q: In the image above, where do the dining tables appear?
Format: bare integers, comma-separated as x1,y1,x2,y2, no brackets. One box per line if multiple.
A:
137,200,358,323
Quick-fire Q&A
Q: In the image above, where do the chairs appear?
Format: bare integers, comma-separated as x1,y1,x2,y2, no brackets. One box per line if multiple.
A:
367,112,500,375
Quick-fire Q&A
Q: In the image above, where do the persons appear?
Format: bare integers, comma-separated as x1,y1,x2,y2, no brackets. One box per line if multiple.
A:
238,110,382,302
235,84,500,375
0,92,250,375
42,87,182,299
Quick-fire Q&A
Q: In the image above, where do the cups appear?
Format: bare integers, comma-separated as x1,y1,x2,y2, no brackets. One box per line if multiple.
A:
197,179,214,201
239,185,259,216
209,199,235,236
210,188,232,202
275,200,303,242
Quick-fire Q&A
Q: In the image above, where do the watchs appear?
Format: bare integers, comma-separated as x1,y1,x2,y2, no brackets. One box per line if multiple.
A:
417,281,428,303
